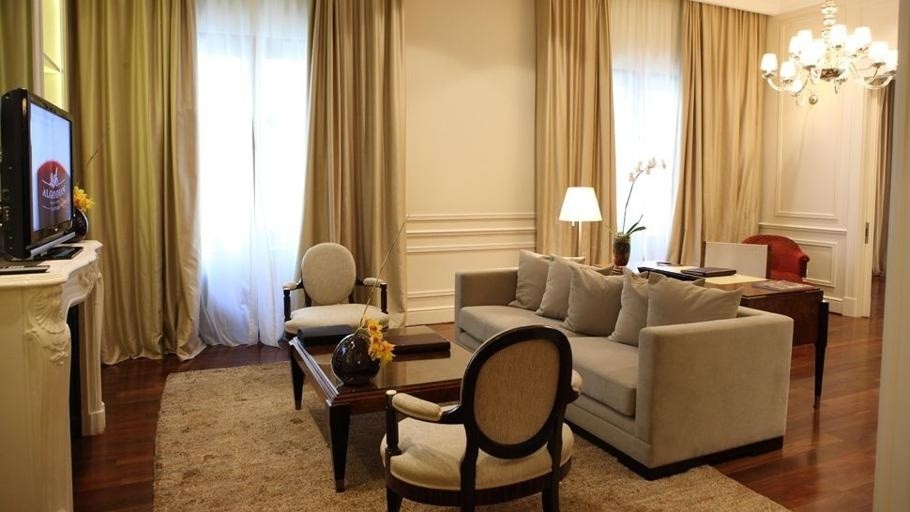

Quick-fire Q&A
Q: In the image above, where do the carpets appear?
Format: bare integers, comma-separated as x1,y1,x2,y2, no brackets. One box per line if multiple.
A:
148,359,793,511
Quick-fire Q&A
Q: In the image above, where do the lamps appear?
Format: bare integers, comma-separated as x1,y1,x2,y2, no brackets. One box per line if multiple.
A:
558,183,608,257
758,0,901,99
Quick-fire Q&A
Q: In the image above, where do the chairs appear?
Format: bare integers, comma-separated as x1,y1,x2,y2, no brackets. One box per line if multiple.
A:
741,234,815,286
375,321,583,511
278,238,391,410
697,239,773,279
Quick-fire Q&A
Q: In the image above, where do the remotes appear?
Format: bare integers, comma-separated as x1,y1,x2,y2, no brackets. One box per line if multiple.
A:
0,265,49,273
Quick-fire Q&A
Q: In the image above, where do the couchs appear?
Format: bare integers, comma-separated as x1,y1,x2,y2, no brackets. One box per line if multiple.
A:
454,267,797,482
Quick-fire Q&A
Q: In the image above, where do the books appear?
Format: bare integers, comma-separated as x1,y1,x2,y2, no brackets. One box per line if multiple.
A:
680,266,737,277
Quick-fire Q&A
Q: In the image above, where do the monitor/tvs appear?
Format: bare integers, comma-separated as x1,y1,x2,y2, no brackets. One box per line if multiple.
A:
0,88,83,262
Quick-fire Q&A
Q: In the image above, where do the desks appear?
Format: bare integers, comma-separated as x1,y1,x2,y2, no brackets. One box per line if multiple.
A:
638,264,831,410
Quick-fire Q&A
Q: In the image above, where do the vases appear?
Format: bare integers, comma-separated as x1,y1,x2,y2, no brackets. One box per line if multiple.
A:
612,235,633,267
329,332,382,387
73,208,90,240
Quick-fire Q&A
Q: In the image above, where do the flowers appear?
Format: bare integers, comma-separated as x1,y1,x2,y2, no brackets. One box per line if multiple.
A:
604,156,669,240
71,144,101,214
355,219,399,369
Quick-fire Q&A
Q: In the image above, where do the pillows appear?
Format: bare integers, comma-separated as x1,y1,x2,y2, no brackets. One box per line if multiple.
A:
644,270,745,329
532,248,616,322
604,263,706,349
506,246,585,315
560,256,648,337
